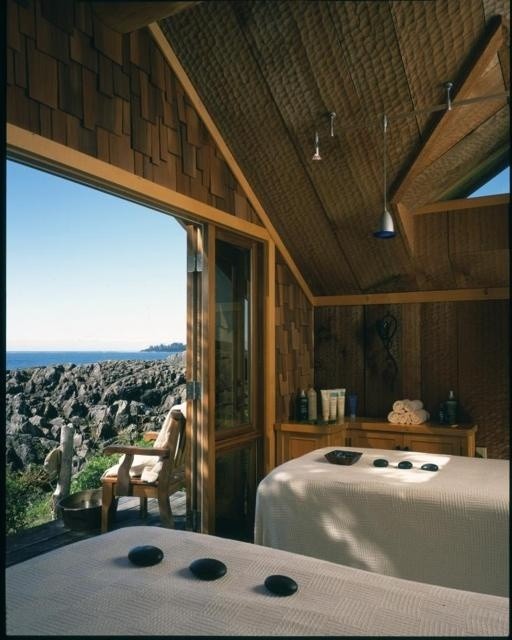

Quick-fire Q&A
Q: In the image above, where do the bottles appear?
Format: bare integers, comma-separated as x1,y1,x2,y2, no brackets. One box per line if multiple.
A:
296,389,308,421
306,384,318,421
445,389,459,426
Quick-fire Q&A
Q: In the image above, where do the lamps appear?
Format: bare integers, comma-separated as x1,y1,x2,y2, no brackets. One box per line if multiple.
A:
372,116,397,239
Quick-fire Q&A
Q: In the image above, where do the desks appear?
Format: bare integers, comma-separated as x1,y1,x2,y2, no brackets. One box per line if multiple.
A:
5,526,509,637
254,445,511,598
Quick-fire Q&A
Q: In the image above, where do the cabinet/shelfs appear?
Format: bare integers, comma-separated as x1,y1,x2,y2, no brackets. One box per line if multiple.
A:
274,416,478,468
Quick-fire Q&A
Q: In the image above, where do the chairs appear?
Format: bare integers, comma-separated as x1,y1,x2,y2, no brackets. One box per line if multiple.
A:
100,411,191,533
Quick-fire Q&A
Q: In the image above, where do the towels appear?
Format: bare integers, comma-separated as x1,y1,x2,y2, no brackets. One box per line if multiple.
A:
387,398,430,425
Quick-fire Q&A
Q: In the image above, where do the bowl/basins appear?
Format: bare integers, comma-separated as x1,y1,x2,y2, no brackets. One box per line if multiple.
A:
56,487,122,531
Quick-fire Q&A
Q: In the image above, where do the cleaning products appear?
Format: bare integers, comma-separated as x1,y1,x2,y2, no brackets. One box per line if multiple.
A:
306,383,317,419
298,388,307,418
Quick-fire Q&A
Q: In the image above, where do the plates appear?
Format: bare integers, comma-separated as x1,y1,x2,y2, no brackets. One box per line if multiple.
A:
324,450,363,466
